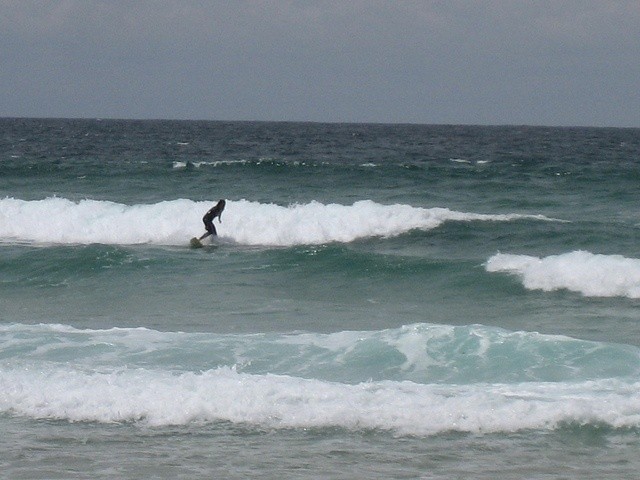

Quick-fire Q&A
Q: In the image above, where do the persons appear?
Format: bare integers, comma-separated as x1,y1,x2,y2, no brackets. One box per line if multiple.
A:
199,200,225,241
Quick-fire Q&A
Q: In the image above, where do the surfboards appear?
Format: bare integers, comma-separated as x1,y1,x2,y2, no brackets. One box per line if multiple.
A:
190,237,202,248
